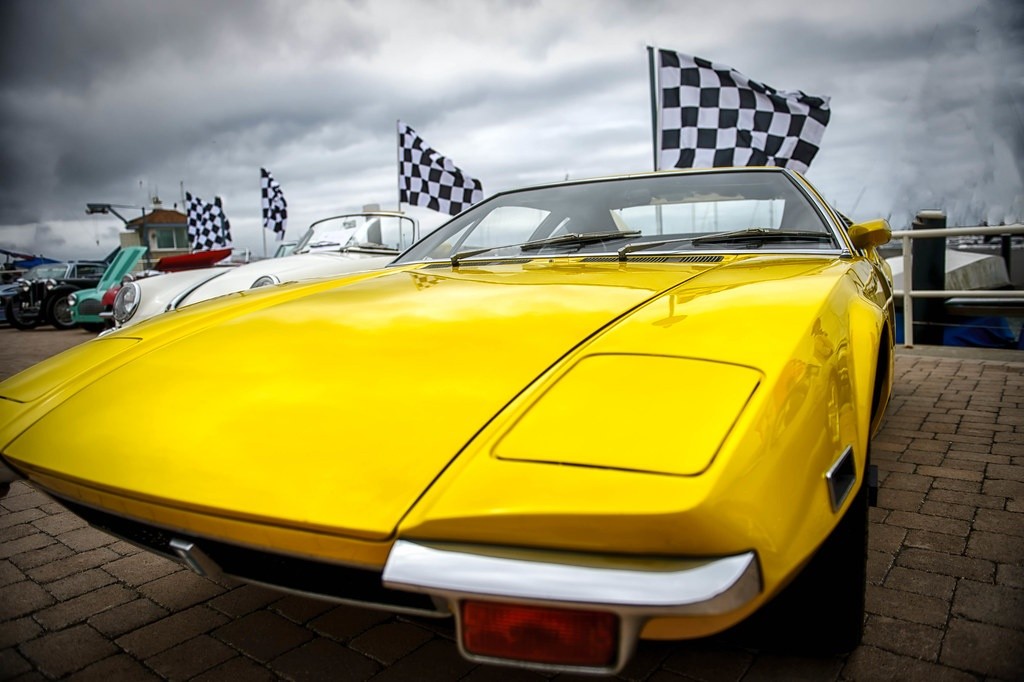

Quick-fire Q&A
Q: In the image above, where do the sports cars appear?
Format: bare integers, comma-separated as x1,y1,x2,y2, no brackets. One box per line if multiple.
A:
0,207,470,335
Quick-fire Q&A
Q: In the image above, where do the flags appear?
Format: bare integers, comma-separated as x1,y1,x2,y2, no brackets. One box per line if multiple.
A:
399,123,484,216
185,192,232,250
658,48,831,175
261,168,288,241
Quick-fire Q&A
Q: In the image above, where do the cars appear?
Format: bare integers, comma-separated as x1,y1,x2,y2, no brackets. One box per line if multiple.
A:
0,164,898,682
